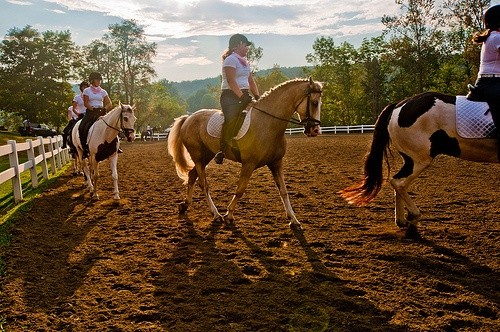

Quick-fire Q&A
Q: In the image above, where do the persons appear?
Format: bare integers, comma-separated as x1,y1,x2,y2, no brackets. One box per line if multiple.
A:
79,72,124,159
215,33,260,164
146,123,153,136
472,4,500,162
68,81,90,154
61,97,76,149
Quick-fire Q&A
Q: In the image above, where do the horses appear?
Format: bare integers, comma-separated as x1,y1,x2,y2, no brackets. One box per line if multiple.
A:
66,99,138,203
167,76,324,231
337,91,500,237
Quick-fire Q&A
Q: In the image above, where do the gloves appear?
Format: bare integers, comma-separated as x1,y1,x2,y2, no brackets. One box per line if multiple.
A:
255,95,260,100
239,93,252,107
79,113,84,116
100,108,106,114
92,107,99,115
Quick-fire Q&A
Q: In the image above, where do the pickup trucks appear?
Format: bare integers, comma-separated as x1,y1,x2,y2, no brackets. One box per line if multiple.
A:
19,123,58,139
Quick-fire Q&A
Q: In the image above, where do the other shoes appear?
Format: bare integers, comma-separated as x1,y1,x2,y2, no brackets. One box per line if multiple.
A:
61,145,67,149
214,150,225,164
83,150,87,158
69,149,73,154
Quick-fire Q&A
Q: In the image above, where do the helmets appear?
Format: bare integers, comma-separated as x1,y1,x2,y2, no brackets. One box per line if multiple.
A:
89,72,102,82
229,34,252,49
72,100,75,103
148,124,150,125
80,82,89,88
485,5,500,29
